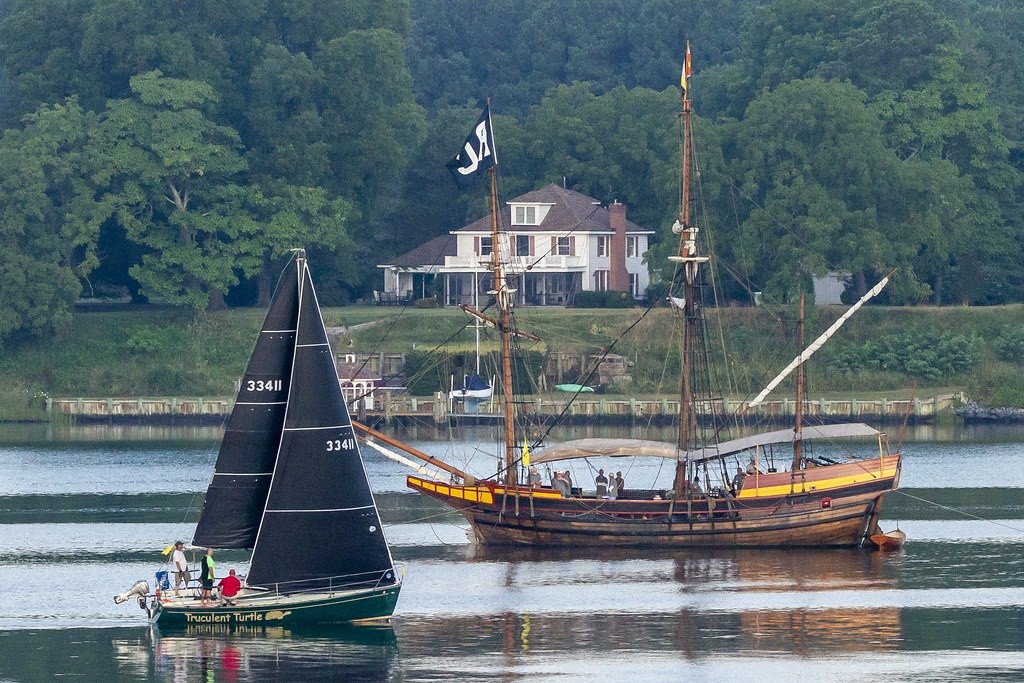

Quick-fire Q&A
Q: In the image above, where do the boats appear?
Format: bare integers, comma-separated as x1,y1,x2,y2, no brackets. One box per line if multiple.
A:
449,374,493,402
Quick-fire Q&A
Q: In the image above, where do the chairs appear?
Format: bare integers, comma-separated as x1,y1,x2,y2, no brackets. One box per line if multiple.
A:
373,290,414,306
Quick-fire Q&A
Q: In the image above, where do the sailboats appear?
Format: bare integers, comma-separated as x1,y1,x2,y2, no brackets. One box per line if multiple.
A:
114,247,408,632
406,34,903,550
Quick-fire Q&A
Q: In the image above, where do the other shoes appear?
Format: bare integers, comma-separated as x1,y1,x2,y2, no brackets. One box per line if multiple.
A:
230,602,237,606
218,603,228,606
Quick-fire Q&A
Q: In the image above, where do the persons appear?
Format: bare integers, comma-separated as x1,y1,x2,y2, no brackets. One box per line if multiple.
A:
173,541,188,598
608,471,624,500
596,469,608,495
691,476,705,499
200,548,215,608
528,468,542,488
732,467,747,498
551,471,572,498
217,569,241,606
746,457,757,475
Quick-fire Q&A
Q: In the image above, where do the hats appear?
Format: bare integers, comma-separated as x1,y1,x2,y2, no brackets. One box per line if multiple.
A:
175,541,184,546
609,473,615,476
230,569,235,574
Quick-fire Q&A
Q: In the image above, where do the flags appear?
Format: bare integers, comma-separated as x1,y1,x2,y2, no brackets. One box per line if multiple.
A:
445,104,498,193
